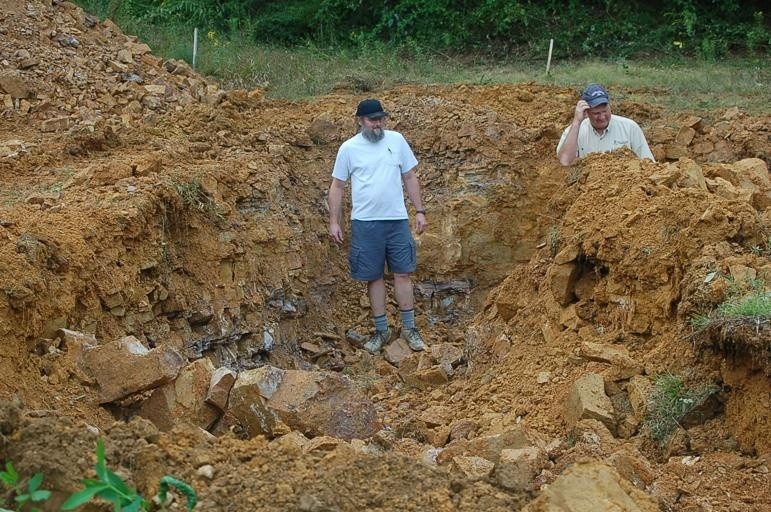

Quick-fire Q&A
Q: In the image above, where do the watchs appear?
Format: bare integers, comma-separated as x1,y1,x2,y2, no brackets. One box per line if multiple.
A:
415,209,425,215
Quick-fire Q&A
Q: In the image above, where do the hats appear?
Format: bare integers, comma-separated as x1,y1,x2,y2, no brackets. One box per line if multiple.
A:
582,85,609,109
358,99,388,119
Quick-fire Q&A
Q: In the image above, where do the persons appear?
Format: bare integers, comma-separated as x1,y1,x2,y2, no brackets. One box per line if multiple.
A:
555,83,656,166
328,98,427,354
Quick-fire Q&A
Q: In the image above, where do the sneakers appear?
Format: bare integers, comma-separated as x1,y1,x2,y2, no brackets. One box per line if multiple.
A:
363,326,392,352
399,327,425,351
